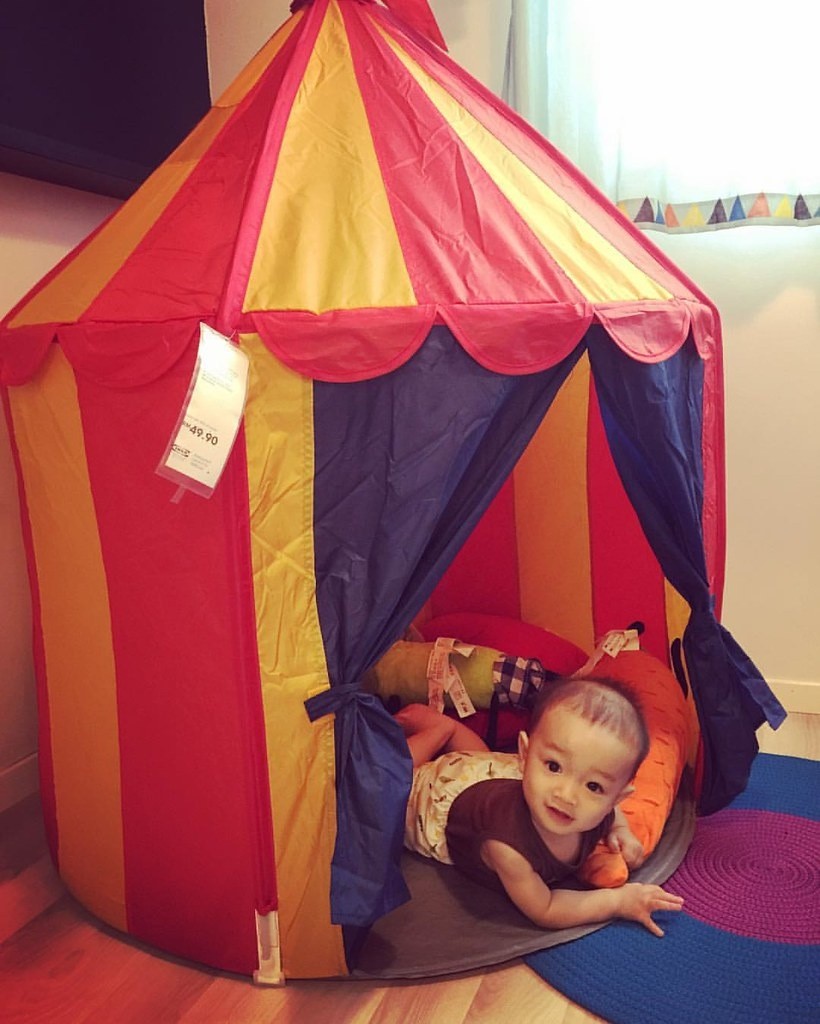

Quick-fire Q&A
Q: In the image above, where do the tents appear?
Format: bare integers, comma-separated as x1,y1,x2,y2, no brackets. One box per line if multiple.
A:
3,1,787,983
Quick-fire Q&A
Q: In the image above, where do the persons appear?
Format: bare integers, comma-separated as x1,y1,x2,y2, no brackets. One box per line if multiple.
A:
391,672,685,937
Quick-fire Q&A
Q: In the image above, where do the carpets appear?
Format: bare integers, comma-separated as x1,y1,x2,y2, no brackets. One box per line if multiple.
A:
525,751,820,1024
